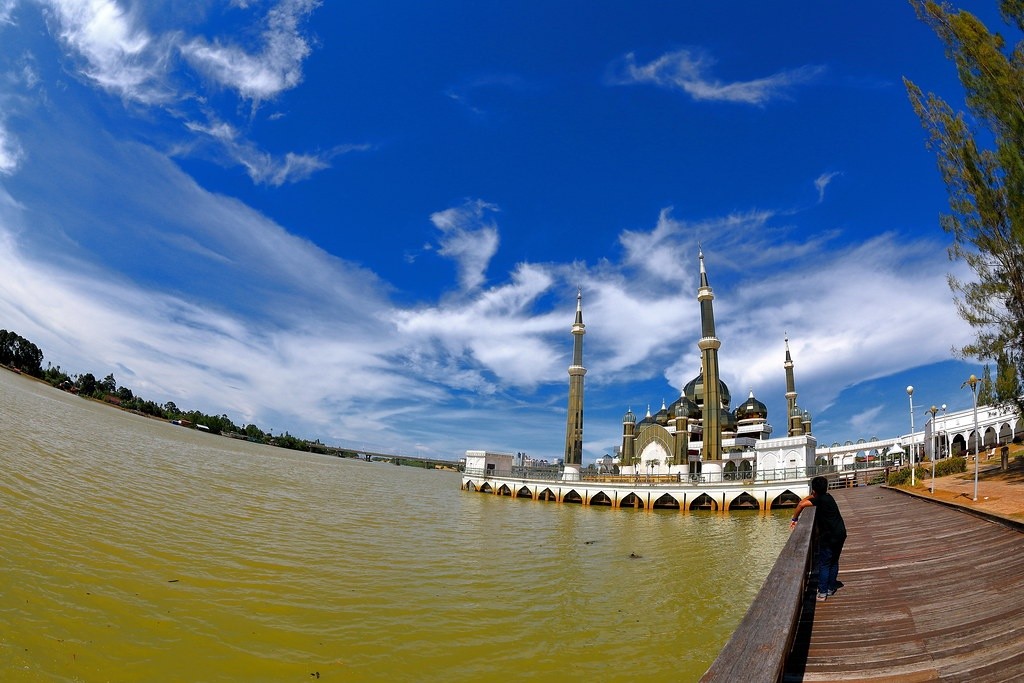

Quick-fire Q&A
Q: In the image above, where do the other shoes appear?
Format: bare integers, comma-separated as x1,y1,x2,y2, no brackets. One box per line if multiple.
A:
806,592,827,603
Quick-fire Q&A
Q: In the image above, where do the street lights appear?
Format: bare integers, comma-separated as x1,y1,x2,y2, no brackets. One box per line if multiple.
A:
960,375,986,502
924,405,939,494
940,404,948,461
907,386,915,486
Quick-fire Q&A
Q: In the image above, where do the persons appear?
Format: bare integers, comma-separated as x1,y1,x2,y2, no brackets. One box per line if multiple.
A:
915,453,919,462
920,450,925,462
999,443,1009,471
677,471,681,482
789,476,847,601
635,471,639,482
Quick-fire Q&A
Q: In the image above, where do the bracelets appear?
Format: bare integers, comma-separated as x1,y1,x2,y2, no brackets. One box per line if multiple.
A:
791,518,798,521
